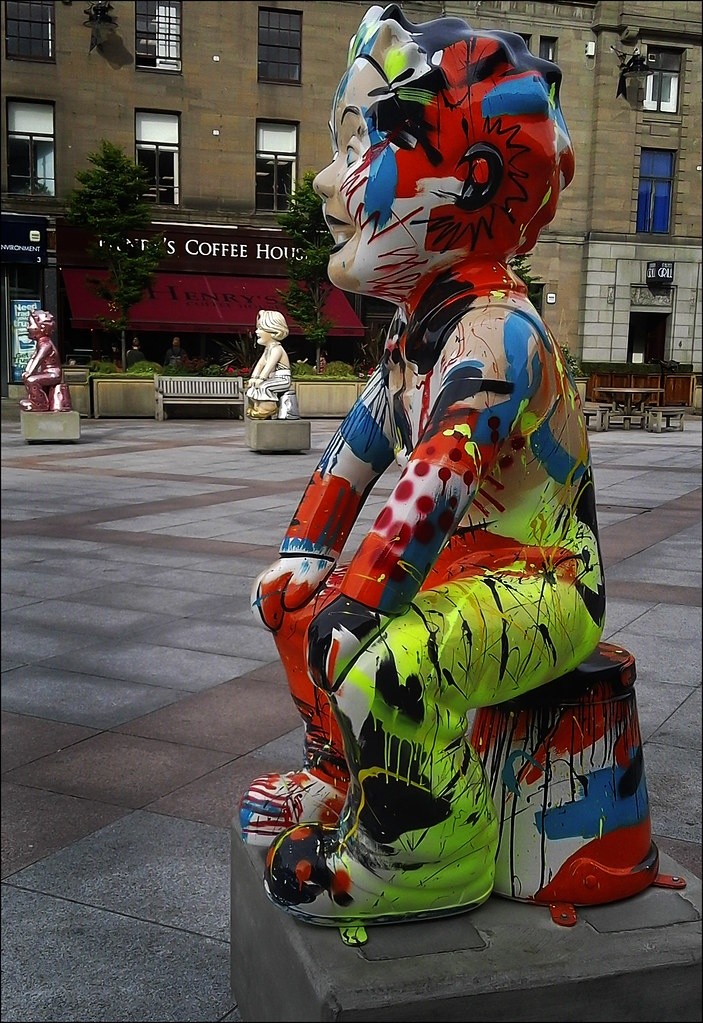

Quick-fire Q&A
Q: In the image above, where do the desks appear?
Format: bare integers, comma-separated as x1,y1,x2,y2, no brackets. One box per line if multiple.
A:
594,388,665,430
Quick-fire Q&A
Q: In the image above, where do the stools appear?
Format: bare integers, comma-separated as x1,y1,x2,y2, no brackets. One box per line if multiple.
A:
582,402,686,433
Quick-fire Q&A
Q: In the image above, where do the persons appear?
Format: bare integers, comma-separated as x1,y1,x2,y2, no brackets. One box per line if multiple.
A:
19,310,61,412
236,4,606,928
163,337,187,366
126,337,146,367
245,310,291,419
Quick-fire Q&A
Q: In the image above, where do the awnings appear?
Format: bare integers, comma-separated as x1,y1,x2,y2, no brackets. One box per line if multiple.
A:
61,269,369,337
